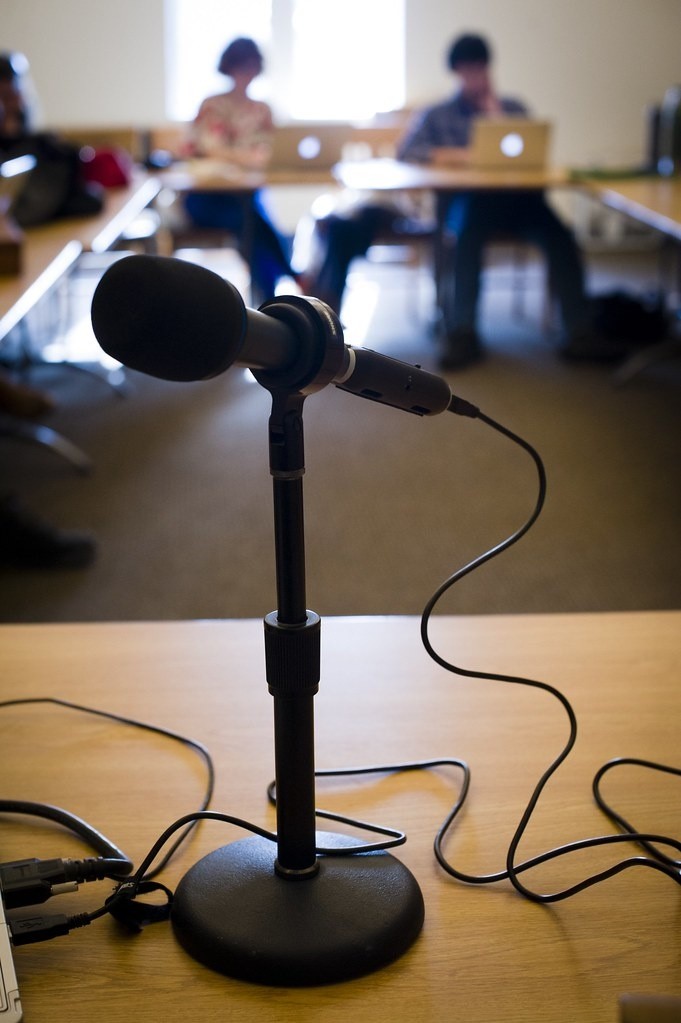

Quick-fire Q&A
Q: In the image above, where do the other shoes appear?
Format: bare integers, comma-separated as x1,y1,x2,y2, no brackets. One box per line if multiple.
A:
295,270,318,295
438,341,487,373
565,355,621,383
0,493,101,577
0,380,62,419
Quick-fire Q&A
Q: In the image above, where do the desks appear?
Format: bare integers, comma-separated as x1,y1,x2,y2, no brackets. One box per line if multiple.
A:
0,155,681,478
1,609,680,1023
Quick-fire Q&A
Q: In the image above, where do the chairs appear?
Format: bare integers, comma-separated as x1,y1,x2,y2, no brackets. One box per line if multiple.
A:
402,109,551,332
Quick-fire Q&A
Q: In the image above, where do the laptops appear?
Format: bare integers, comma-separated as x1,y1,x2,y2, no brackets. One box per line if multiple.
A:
465,119,554,170
0,155,39,217
240,124,358,172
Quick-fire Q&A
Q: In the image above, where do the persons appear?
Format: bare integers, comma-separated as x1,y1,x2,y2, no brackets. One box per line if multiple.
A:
399,35,586,372
182,38,306,307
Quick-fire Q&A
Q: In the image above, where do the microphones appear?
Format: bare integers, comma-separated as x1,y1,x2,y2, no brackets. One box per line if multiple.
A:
89,255,481,421
